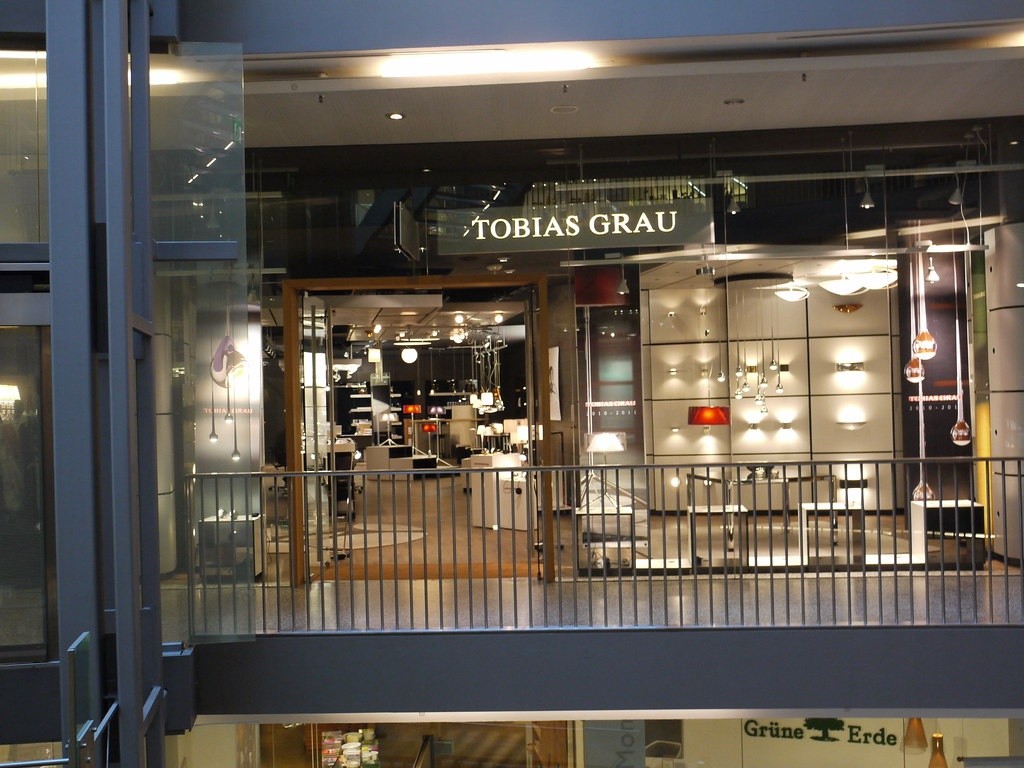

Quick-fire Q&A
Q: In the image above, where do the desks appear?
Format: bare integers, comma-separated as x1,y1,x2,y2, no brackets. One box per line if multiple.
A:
390,456,438,481
461,458,472,493
476,433,513,454
471,453,537,530
367,446,412,482
686,473,837,551
304,438,356,520
198,515,265,580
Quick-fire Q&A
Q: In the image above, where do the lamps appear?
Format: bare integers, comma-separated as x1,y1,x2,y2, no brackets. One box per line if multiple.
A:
671,426,681,434
667,311,675,318
949,230,971,447
266,524,431,554
860,179,874,209
572,264,647,508
333,327,528,480
728,200,741,214
822,268,897,430
900,716,948,768
904,223,941,500
667,369,680,377
204,277,220,444
688,280,809,434
221,285,242,461
947,175,965,206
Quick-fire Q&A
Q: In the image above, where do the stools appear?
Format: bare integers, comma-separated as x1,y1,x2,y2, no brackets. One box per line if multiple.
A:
574,510,635,576
911,500,984,564
687,504,750,570
798,504,864,569
